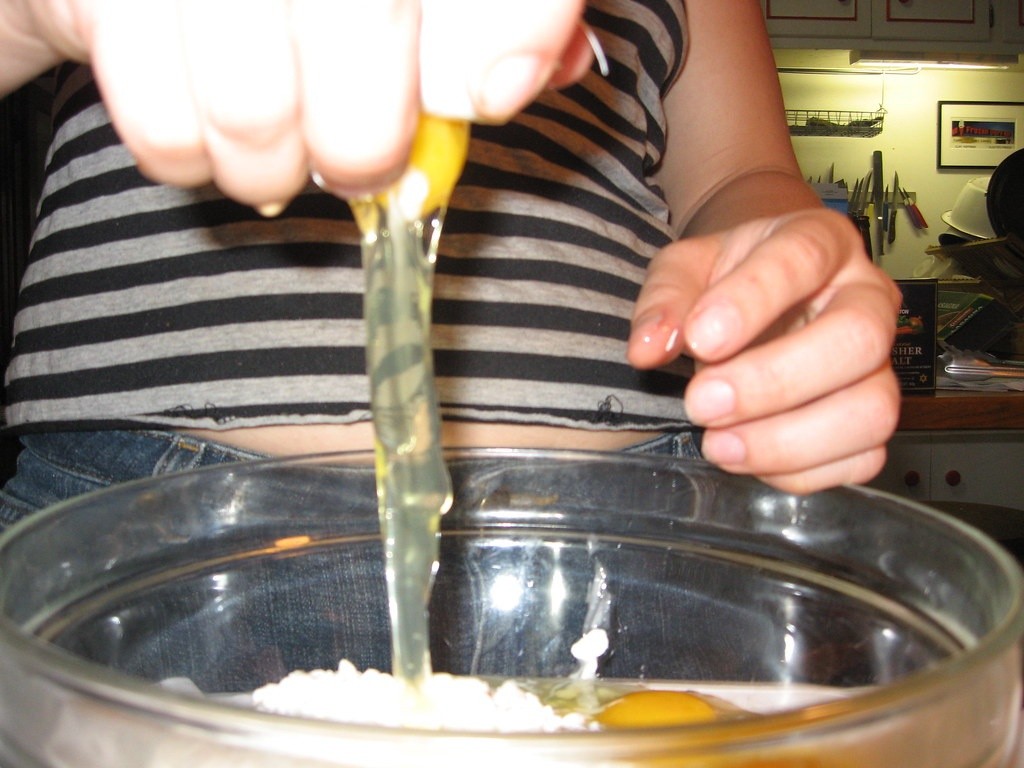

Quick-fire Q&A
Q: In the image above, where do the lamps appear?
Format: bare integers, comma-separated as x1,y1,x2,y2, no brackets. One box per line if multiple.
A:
848,50,1018,69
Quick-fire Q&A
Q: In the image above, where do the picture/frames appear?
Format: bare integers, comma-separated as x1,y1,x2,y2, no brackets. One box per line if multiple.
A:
937,100,1024,169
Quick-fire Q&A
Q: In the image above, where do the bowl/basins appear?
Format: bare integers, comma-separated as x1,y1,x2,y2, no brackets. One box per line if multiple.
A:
1,448,1024,767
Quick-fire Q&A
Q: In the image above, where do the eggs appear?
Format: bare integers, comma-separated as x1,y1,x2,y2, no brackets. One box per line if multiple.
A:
336,116,470,681
554,682,769,731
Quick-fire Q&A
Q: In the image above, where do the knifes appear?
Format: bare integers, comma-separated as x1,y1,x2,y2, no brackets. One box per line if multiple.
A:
888,170,899,244
847,168,873,230
806,162,834,183
884,184,888,232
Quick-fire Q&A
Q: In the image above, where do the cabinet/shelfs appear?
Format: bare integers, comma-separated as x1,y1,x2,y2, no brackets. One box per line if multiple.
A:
759,0,1024,52
865,390,1024,507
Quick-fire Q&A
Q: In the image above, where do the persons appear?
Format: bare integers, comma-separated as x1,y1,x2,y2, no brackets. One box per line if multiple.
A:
0,0,903,694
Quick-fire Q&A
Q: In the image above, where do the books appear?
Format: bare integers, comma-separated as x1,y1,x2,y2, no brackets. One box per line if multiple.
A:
951,367,1024,377
